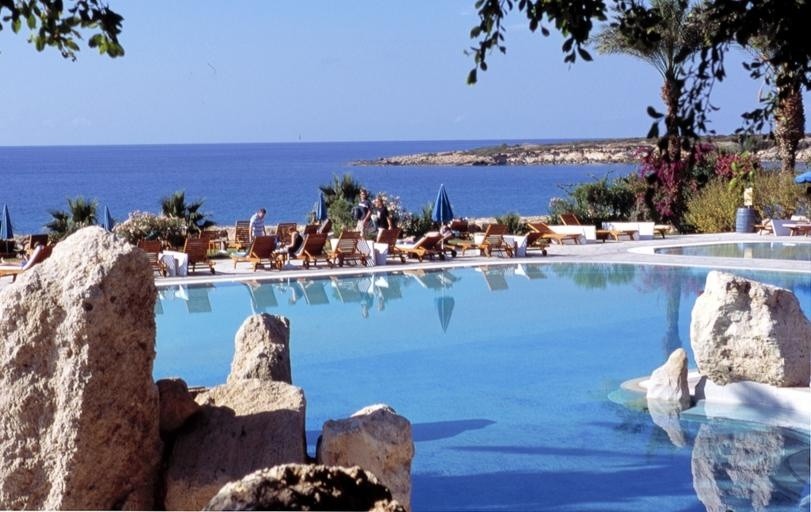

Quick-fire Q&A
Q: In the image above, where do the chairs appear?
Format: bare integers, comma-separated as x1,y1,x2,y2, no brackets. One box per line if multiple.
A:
527,213,640,245
230,218,550,271
0,231,215,283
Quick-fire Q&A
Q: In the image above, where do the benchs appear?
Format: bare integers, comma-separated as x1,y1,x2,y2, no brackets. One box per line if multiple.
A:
783,221,811,236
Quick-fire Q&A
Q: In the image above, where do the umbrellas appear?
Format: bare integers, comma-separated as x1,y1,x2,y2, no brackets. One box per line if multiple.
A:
432,270,456,334
1,201,13,252
316,192,327,227
431,184,453,250
794,171,811,183
103,204,113,232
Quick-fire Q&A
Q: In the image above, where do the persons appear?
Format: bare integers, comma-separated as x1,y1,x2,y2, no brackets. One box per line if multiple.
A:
0,237,59,271
248,208,267,248
250,298,266,315
287,286,303,305
360,285,385,319
793,186,811,217
352,187,390,241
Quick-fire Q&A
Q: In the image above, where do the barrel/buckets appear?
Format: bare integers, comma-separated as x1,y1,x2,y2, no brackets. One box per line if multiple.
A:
736,207,754,233
